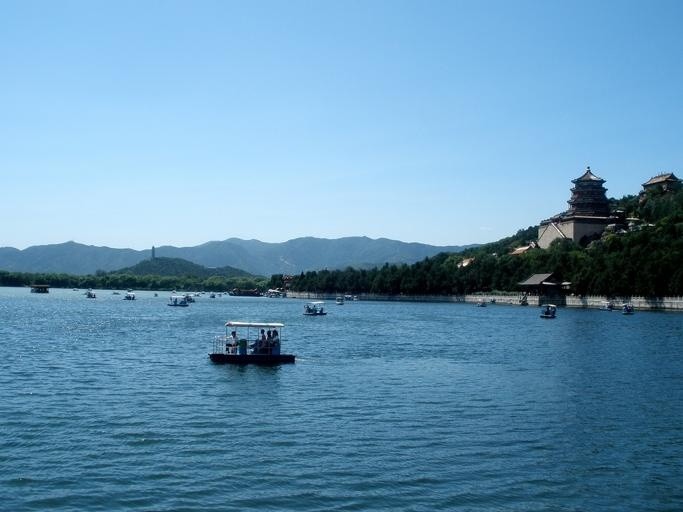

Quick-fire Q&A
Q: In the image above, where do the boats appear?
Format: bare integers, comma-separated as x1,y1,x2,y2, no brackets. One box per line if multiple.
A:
72,288,177,297
166,296,189,307
29,285,51,294
207,321,296,365
538,304,556,318
85,292,96,298
621,303,634,314
302,301,326,315
183,295,195,303
489,300,495,304
475,301,486,307
599,301,619,312
344,295,359,301
192,287,287,299
335,296,343,305
121,293,138,300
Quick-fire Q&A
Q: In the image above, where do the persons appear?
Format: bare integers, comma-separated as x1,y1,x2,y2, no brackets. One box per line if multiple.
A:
248,329,279,354
225,330,237,352
